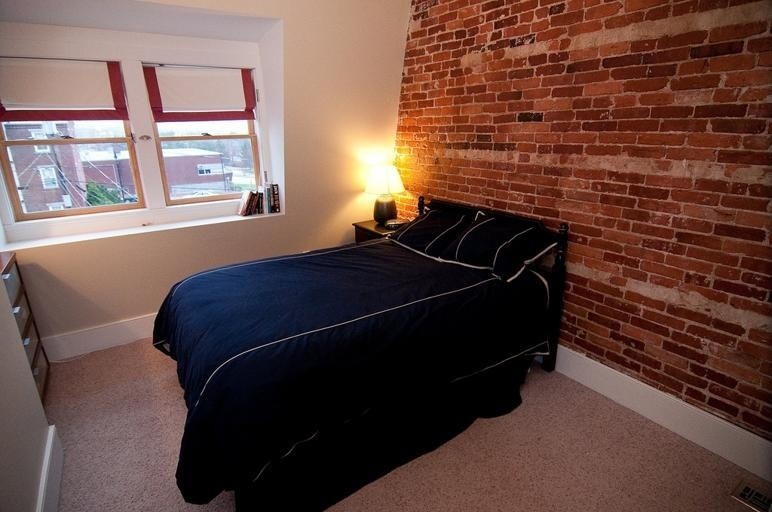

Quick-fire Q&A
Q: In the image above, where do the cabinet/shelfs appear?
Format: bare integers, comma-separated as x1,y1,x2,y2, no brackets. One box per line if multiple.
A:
0,251,50,407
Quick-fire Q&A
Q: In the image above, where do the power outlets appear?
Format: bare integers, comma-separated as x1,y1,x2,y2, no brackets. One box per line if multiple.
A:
731,481,772,512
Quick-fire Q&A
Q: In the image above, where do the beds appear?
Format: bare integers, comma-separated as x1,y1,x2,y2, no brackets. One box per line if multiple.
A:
152,195,569,512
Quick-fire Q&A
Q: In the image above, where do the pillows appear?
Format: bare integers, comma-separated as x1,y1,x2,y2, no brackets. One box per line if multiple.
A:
385,207,469,260
440,210,558,283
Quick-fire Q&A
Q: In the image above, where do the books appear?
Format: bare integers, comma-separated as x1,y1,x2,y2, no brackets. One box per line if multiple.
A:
236,182,281,216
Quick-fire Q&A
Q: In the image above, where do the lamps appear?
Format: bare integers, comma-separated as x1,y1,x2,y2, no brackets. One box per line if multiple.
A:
366,165,405,225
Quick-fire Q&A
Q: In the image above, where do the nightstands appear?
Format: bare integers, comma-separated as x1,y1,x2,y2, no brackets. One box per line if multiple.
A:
352,219,406,243
